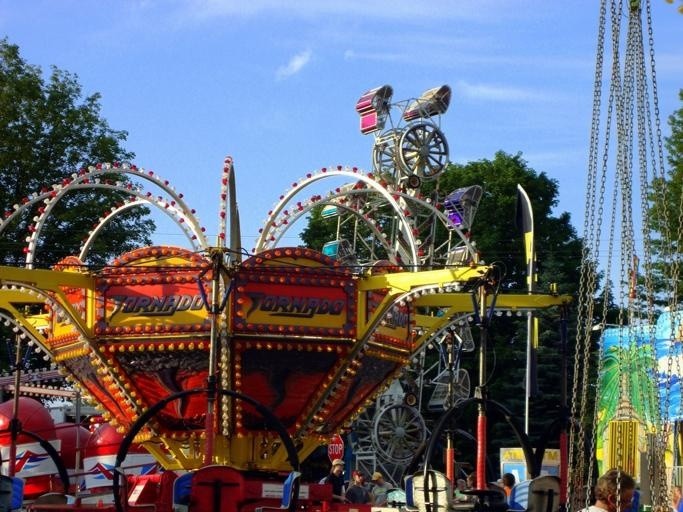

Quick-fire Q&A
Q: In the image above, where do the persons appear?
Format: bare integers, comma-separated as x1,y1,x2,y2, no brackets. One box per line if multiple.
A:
668,485,683,511
371,471,393,505
343,470,370,504
453,478,469,503
466,472,477,493
576,468,637,512
499,472,516,506
322,458,347,504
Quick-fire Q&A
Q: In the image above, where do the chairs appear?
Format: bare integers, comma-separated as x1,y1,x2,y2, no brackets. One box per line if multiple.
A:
1,465,561,512
